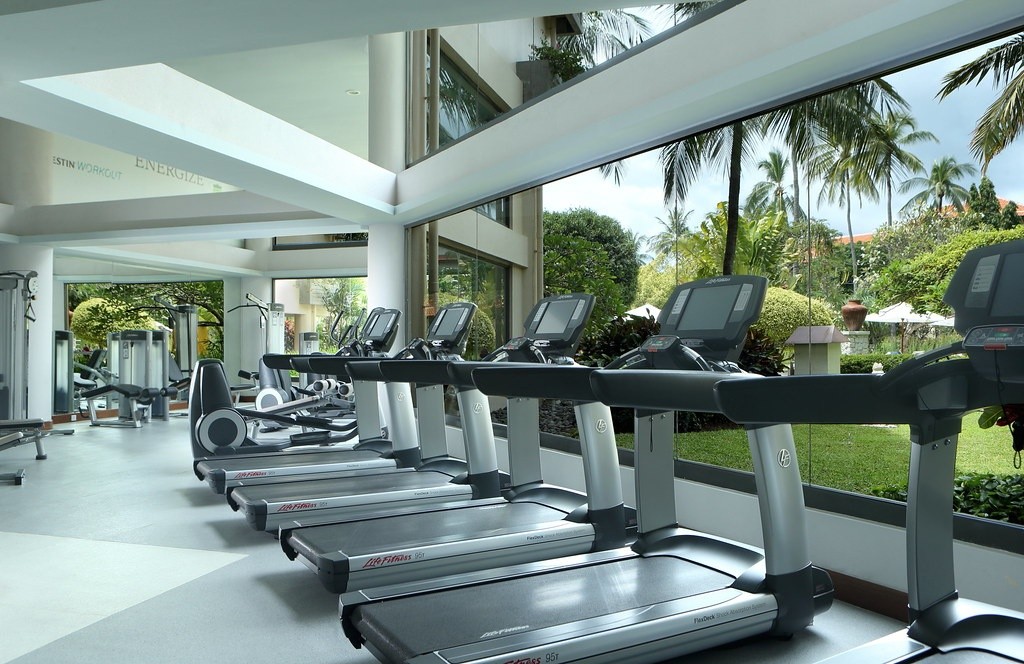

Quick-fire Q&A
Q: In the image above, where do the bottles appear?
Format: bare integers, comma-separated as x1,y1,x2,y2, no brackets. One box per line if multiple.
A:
841,299,868,330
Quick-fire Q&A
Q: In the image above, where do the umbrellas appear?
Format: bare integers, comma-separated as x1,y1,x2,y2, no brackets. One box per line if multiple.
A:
865,301,955,355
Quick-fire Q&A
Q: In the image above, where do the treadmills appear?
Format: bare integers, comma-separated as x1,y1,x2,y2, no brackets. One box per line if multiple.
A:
276,293,636,595
224,302,511,542
713,238,1024,664
338,275,835,664
192,308,422,494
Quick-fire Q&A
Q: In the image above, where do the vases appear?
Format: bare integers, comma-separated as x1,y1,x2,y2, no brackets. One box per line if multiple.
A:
841,300,867,330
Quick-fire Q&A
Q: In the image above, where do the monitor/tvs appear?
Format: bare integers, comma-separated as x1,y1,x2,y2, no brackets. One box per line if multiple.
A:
991,252,1024,316
369,314,392,336
676,284,740,330
434,309,464,335
535,300,577,334
